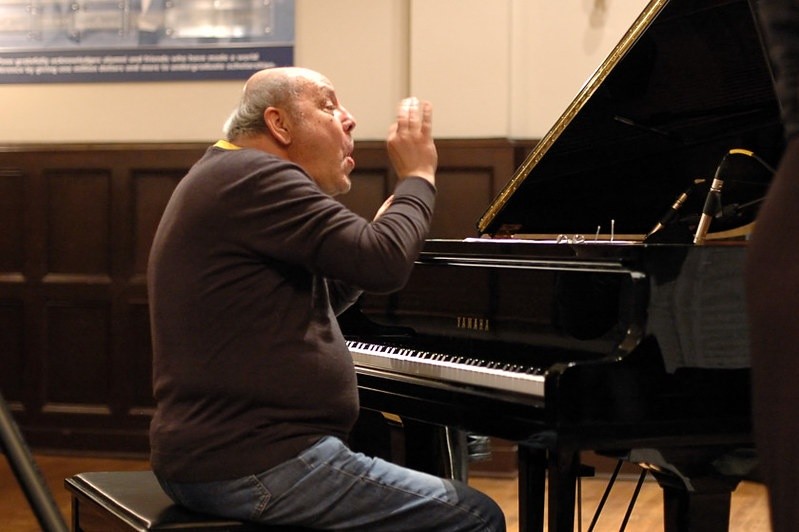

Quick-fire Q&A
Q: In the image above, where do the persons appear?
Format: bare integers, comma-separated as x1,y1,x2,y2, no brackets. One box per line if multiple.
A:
148,66,506,532
743,140,799,532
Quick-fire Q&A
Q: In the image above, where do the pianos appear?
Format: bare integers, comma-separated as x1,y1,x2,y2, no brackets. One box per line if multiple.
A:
334,0,798,532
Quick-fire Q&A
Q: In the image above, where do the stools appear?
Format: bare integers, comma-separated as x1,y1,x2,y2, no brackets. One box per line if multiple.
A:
64,470,242,532
